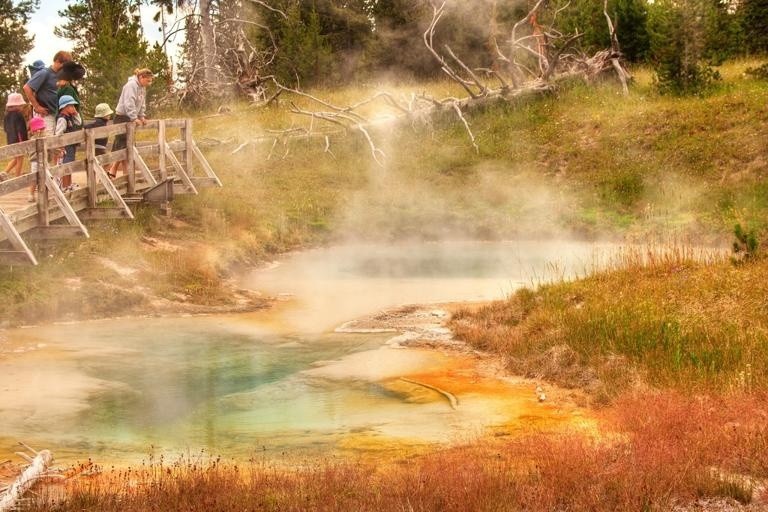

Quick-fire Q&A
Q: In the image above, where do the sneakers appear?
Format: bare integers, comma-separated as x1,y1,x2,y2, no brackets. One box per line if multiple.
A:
0,170,81,202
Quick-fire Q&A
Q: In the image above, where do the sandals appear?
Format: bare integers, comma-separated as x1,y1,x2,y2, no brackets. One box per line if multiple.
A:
107,172,116,180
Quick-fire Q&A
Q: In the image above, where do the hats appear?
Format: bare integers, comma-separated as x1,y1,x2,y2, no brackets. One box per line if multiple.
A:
58,95,80,111
26,60,45,70
28,116,47,132
55,61,85,81
94,103,114,118
5,93,26,106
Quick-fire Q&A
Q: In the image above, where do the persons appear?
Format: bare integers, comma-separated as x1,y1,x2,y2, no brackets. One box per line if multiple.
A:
55,95,84,193
84,102,115,180
22,49,74,189
56,65,82,129
0,90,28,182
106,68,154,177
25,114,48,204
